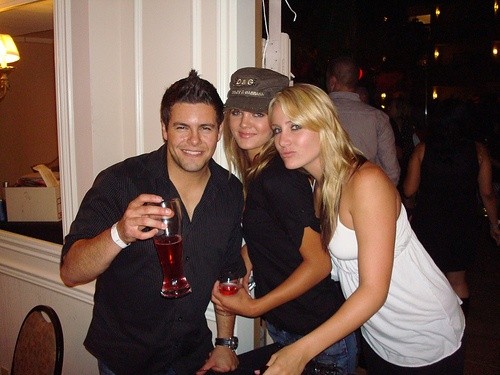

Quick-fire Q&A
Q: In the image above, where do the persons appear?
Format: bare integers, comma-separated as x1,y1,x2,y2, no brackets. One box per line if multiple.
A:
390,95,499,301
326,56,401,191
59,68,247,375
204,66,360,375
263,83,468,374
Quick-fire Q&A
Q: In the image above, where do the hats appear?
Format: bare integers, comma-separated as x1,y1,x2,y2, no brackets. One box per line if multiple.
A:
224,67,289,114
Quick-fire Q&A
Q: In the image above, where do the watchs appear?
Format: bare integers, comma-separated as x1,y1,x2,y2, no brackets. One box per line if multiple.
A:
215,336,239,352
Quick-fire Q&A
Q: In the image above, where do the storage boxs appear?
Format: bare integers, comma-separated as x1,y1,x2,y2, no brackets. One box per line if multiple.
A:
4,163,62,222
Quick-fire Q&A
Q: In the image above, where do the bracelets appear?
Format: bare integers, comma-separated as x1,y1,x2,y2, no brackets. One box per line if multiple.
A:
110,221,131,249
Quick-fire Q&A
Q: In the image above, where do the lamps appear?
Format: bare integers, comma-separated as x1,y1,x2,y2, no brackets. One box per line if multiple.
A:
0,34,21,100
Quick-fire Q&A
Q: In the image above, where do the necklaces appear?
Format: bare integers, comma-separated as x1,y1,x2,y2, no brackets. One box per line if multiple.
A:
307,176,316,193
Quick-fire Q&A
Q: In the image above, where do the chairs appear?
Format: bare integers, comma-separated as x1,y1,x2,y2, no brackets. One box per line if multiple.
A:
191,342,286,375
11,304,65,375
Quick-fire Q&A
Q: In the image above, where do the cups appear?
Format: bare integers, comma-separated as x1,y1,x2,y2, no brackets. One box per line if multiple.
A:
146,198,192,298
218,272,240,295
